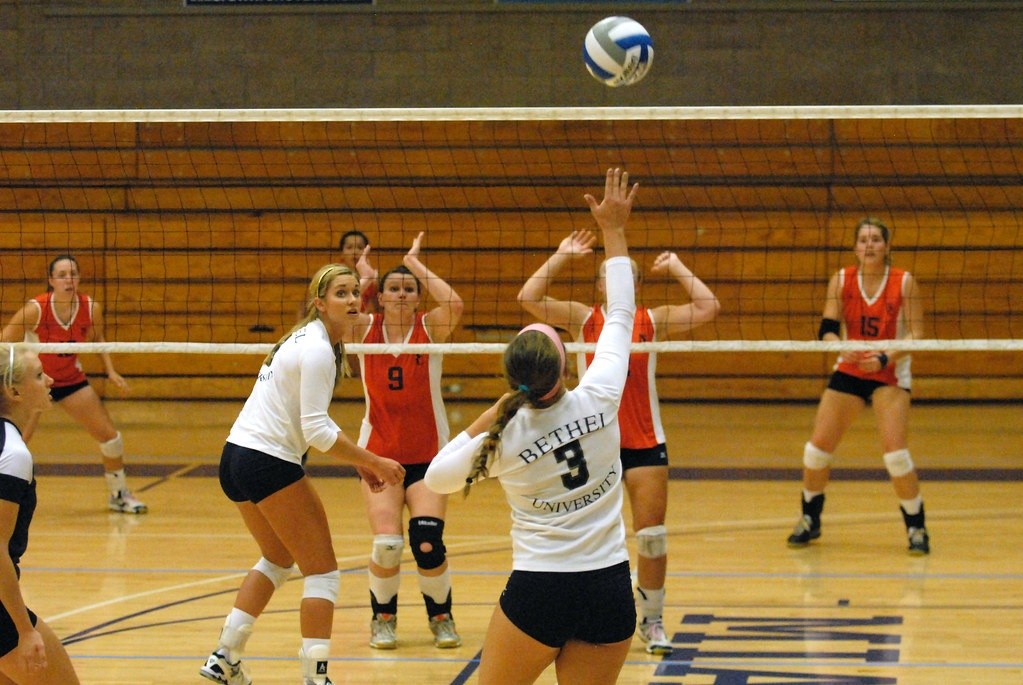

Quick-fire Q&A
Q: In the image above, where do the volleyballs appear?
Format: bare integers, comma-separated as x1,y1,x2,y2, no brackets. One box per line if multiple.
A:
582,14,655,88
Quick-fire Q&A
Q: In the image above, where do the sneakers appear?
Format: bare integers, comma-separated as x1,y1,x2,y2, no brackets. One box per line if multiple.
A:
427,614,462,648
368,613,397,649
786,517,819,548
905,527,929,556
304,677,332,685
638,618,673,654
105,493,147,515
198,645,252,685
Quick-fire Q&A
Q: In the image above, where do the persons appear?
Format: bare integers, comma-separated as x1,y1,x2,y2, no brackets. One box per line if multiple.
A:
0,344,81,685
424,167,639,685
0,254,147,513
336,230,375,314
198,263,406,685
355,231,464,649
516,227,721,653
787,219,931,556
